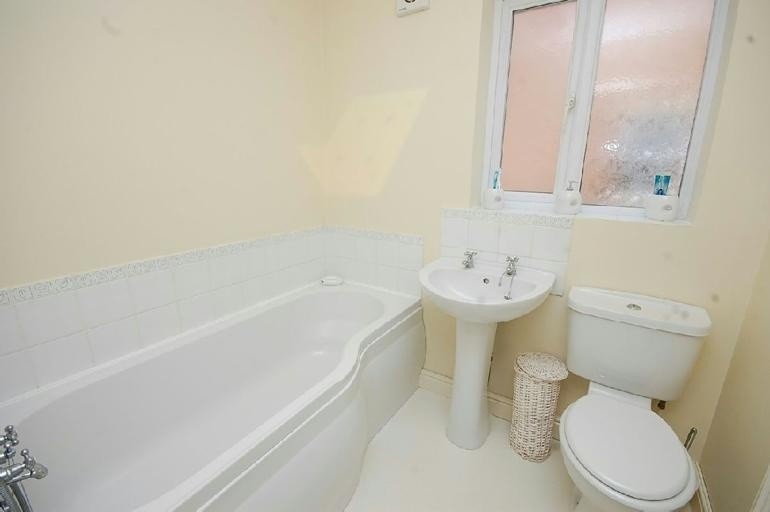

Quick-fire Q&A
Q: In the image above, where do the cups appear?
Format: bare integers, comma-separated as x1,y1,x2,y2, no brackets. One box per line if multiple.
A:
481,188,507,210
643,194,680,222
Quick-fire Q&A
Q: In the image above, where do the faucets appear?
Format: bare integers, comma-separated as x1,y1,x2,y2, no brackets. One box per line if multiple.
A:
461,250,478,268
1,450,50,487
505,255,520,276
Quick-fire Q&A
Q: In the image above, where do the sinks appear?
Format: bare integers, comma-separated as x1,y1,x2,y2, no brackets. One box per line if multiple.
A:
416,255,557,323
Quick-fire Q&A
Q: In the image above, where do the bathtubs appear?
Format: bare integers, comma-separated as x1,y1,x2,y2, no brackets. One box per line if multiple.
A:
1,277,425,512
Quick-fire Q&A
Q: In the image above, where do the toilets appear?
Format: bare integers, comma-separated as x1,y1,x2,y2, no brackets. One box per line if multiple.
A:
558,284,712,512
